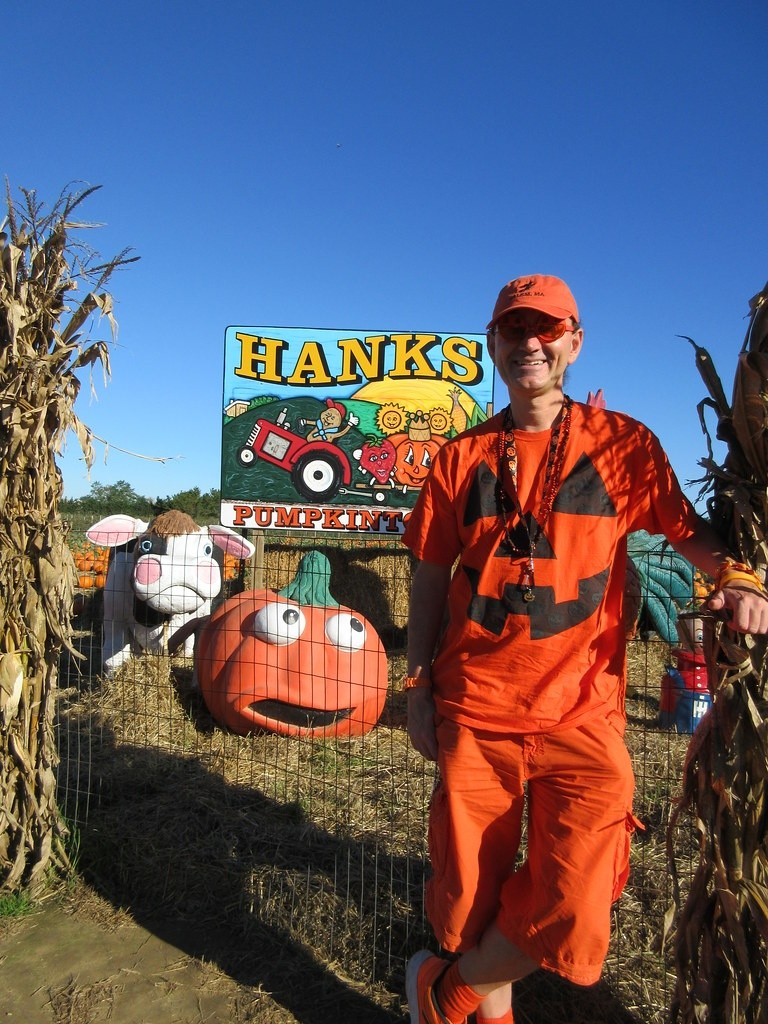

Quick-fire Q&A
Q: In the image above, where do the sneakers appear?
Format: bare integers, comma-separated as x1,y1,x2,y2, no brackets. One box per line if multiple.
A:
405,950,467,1024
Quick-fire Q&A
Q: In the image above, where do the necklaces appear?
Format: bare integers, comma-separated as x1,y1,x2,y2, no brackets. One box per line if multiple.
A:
499,394,572,603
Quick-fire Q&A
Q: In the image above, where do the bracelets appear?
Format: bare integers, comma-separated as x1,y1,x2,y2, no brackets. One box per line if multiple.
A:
403,677,432,689
713,560,765,591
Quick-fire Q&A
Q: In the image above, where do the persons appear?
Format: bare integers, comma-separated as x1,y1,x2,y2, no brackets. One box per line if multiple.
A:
657,608,713,735
405,276,768,1024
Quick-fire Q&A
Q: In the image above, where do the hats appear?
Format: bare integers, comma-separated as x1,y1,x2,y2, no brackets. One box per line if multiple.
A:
486,275,578,329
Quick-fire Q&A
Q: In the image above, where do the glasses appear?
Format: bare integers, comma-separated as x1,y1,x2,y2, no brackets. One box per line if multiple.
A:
495,319,576,343
